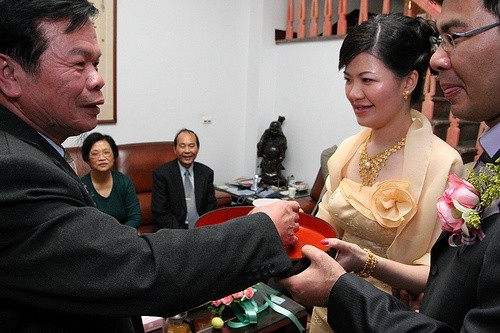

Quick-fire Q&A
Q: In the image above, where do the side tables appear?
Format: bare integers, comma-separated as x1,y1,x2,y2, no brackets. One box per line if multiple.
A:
215,182,312,201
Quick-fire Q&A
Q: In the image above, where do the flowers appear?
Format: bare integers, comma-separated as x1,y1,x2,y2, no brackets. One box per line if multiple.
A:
437,172,486,233
210,286,255,312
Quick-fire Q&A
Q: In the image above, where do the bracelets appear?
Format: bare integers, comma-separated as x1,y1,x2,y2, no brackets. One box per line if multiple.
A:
354,248,378,278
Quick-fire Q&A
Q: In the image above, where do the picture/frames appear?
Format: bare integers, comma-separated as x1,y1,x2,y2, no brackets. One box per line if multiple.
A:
90,0,118,125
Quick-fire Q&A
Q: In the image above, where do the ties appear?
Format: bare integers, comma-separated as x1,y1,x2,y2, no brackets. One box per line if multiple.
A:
183,169,199,229
472,148,500,170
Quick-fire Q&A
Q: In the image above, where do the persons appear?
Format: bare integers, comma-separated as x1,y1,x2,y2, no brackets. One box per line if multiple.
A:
258,121,288,180
0,0,300,333
151,128,218,233
299,13,464,333
274,0,500,333
81,132,143,229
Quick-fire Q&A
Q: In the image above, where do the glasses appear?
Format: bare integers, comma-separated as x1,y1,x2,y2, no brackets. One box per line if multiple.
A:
429,21,500,52
89,149,112,159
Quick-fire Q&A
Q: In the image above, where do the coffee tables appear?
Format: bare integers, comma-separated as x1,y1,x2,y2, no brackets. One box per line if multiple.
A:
143,281,309,333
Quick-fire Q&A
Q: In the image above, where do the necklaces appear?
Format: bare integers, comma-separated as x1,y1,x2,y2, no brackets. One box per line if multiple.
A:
358,130,406,188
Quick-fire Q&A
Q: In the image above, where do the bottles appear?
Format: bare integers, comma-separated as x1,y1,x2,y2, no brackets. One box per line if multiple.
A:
166,314,191,333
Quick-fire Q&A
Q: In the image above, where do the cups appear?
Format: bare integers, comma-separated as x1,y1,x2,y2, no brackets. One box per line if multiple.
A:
288,188,296,198
252,198,280,207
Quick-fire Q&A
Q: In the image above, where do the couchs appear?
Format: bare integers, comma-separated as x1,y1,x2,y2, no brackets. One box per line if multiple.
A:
66,141,177,231
